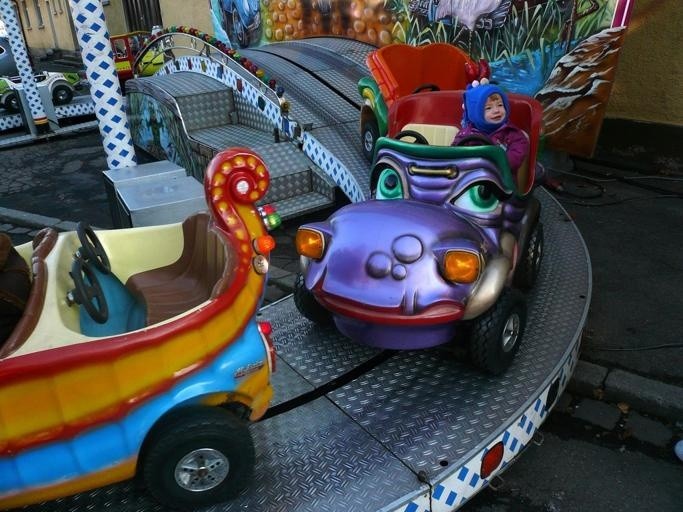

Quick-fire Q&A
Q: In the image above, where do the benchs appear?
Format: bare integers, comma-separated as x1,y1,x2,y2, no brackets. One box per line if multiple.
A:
397,123,530,191
372,42,479,101
124,211,234,328
0,227,58,360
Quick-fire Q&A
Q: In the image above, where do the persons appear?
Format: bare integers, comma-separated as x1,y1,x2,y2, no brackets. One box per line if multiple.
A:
451,78,530,190
